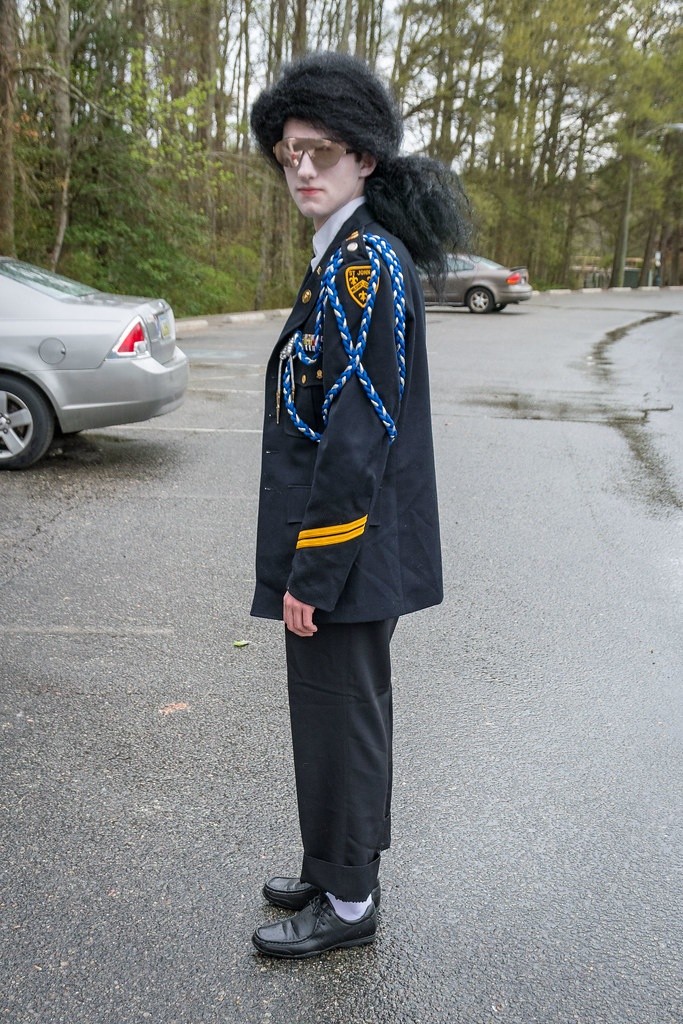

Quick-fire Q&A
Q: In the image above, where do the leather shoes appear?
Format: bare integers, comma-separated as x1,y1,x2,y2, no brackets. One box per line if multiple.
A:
250,894,379,959
262,874,381,911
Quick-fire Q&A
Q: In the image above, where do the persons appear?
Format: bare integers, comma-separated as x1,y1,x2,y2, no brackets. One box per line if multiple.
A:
251,55,468,957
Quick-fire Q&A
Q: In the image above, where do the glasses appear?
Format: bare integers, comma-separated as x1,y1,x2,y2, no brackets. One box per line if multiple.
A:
272,137,366,170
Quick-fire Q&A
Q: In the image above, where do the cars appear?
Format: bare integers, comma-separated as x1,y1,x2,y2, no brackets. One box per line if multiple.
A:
0,256,189,469
414,252,533,314
